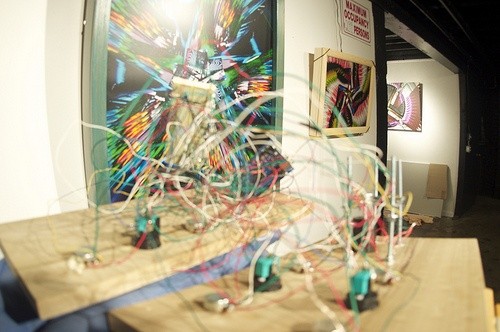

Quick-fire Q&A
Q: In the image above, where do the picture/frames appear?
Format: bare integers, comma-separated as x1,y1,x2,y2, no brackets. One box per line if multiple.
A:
82,0,285,211
308,47,376,136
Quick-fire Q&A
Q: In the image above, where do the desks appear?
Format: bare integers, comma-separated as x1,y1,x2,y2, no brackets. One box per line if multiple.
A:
107,234,491,332
0,183,316,332
